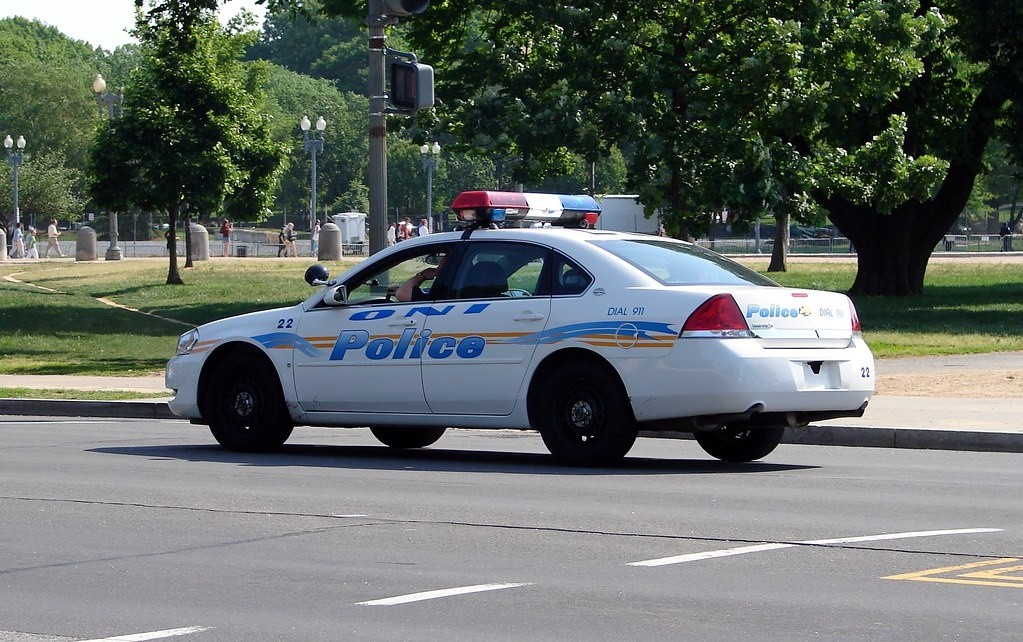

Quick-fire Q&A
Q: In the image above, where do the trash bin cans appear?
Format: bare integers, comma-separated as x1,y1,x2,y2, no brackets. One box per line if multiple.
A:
0,229,7,261
237,245,246,257
318,223,342,261
76,226,96,261
190,224,209,261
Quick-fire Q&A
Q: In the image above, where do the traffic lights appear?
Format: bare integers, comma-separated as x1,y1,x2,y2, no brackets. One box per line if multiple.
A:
392,64,418,109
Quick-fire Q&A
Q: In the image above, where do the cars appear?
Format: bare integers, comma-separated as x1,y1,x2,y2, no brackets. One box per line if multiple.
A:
165,191,875,469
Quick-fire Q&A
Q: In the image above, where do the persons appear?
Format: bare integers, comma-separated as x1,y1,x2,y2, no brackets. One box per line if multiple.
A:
999,223,1013,252
45,220,67,258
278,222,297,257
0,221,38,259
219,218,233,256
659,223,665,237
389,217,428,245
396,256,473,302
310,219,320,257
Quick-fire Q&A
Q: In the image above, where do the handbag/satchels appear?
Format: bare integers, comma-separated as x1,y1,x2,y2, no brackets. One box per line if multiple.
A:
287,230,297,242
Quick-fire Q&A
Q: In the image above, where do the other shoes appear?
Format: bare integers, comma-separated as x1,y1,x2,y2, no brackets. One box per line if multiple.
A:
60,255,67,258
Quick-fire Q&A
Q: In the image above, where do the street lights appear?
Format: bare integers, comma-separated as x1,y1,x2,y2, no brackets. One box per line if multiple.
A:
93,73,124,261
420,142,441,234
301,116,326,240
3,134,26,229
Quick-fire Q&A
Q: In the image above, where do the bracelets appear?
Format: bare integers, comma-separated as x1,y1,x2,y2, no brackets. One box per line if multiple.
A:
416,272,425,281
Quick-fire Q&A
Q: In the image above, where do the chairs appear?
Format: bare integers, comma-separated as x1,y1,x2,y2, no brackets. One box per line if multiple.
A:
461,261,509,298
